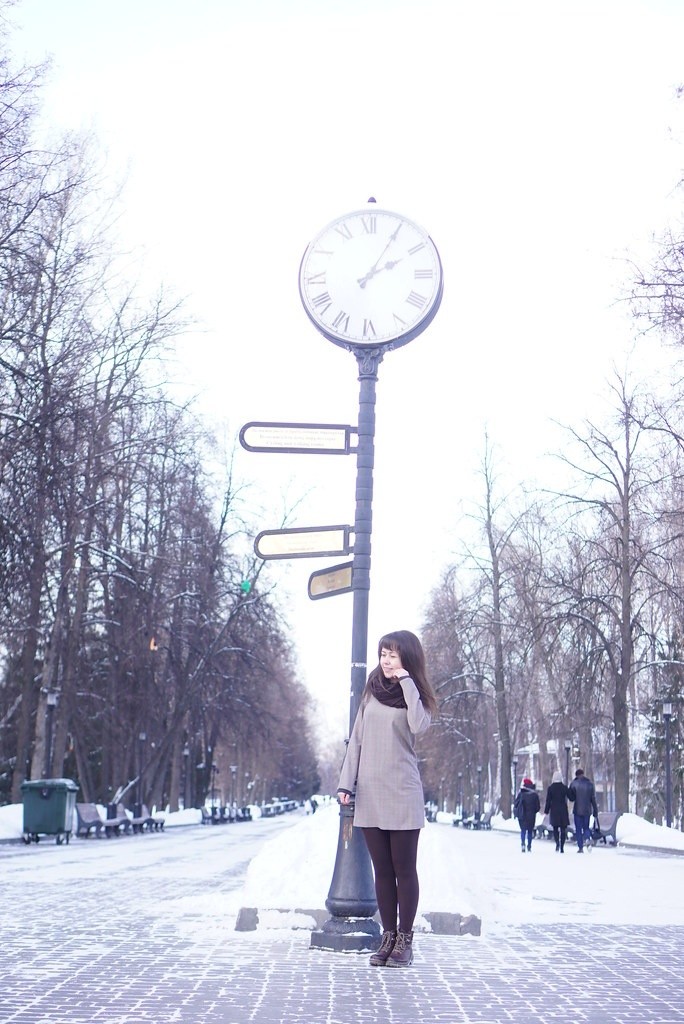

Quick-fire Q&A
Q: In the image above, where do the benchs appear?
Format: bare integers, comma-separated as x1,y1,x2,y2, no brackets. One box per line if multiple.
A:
76,802,166,838
452,810,493,831
201,806,252,823
261,801,299,817
532,811,623,845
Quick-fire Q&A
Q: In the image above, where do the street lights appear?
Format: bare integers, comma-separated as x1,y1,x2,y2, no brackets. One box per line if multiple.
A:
563,737,574,788
457,771,463,816
511,754,519,818
476,765,484,813
656,700,678,827
39,685,63,779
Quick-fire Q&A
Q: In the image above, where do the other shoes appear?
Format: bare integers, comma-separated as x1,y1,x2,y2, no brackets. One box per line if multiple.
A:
527,847,532,852
587,844,592,852
560,848,565,852
576,848,584,853
555,844,560,853
521,846,525,853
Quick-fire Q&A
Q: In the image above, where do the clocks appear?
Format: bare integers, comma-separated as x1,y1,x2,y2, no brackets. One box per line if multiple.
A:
296,209,440,344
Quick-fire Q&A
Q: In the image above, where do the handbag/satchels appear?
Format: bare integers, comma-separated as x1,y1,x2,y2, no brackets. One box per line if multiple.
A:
592,818,606,846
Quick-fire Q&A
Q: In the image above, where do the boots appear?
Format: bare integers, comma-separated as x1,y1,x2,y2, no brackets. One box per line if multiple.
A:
369,930,398,969
386,931,414,968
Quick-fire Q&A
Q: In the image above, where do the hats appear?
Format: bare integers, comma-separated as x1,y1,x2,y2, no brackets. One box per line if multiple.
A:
552,771,562,783
523,779,533,786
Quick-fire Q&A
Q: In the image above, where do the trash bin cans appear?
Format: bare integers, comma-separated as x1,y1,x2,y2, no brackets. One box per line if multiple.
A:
20,778,79,845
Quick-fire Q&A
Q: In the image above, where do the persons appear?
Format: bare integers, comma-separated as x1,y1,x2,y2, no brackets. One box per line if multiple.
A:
305,798,318,814
570,769,598,853
336,630,436,967
544,772,574,853
514,779,540,852
565,814,578,845
533,813,550,839
